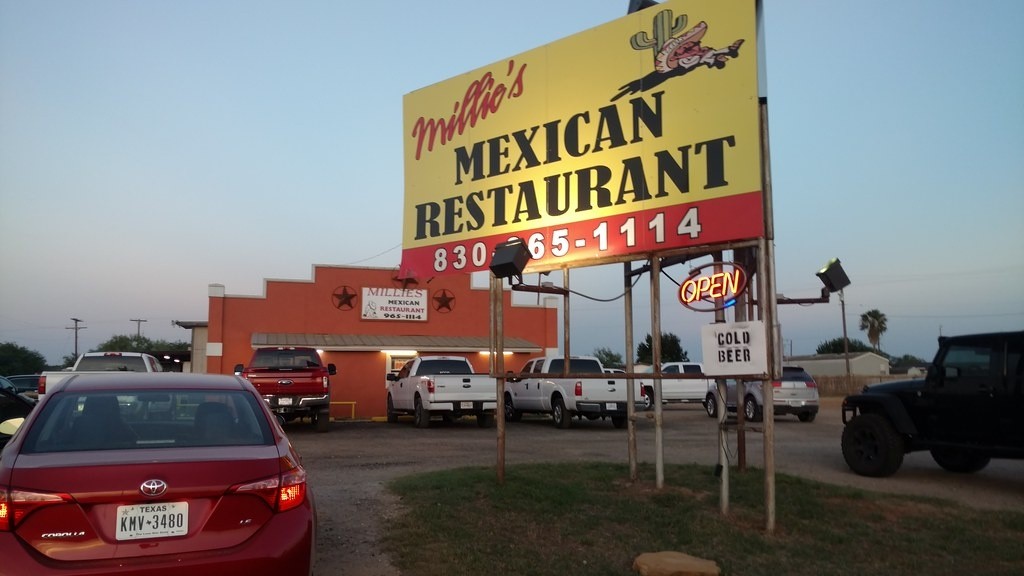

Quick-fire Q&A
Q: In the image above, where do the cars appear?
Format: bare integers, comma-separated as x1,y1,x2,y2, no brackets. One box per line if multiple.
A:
0,373,42,452
706,365,820,423
0,369,320,576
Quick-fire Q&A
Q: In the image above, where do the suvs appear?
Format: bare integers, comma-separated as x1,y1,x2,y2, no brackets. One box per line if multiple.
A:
839,328,1024,477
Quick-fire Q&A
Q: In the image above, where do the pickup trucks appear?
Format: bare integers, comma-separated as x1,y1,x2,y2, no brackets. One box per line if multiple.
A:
38,350,164,416
502,354,648,431
640,360,717,411
233,345,338,434
385,355,499,429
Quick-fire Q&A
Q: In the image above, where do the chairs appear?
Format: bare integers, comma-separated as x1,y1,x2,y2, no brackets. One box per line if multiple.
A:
69,394,133,444
193,401,235,439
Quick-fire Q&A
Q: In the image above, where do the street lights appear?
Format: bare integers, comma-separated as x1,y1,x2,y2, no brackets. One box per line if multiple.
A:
129,318,147,352
71,317,83,361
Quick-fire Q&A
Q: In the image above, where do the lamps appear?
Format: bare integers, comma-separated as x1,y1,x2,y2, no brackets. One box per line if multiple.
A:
489,238,570,295
753,259,850,306
